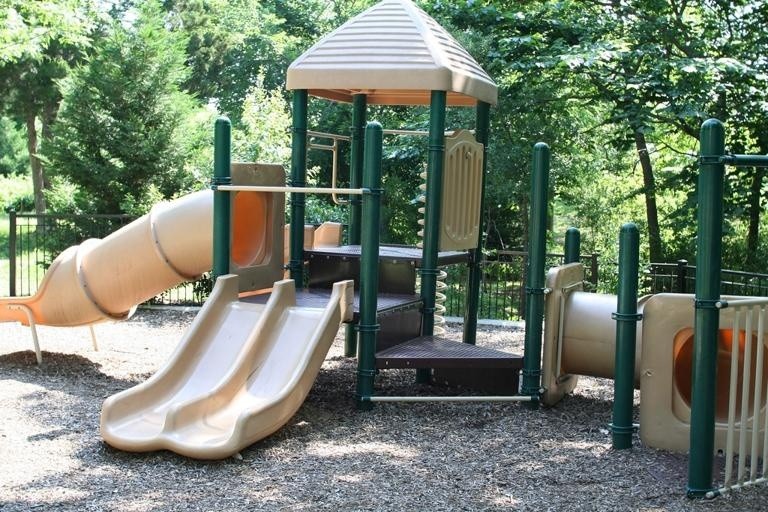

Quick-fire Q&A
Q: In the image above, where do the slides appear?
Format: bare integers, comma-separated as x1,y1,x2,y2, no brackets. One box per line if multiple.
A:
0,188,214,327
100,274,354,458
